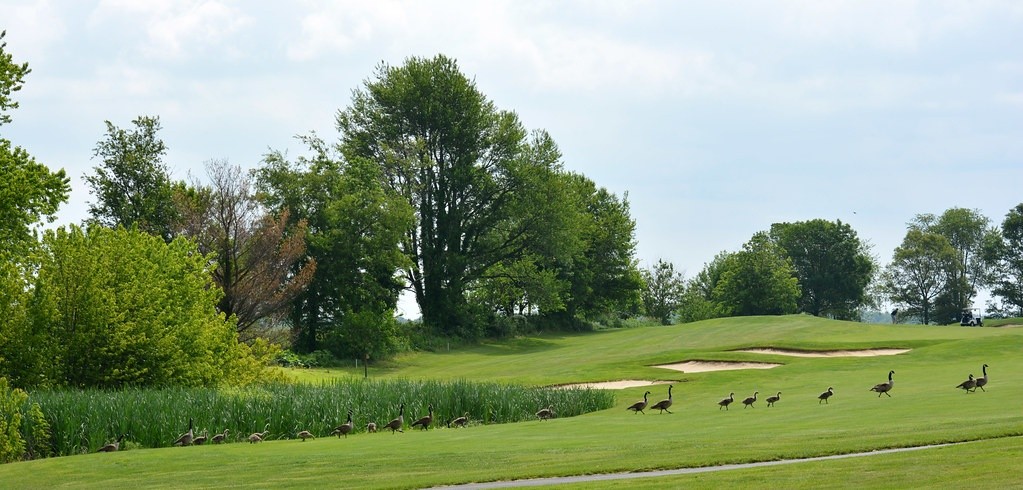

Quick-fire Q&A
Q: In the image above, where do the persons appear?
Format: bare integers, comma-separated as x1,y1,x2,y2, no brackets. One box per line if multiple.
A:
891,308,898,324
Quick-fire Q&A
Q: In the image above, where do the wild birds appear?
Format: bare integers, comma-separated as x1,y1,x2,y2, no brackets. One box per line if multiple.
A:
449,412,469,430
96,432,129,453
869,370,896,398
329,409,354,439
741,391,759,409
383,403,406,435
626,390,652,415
211,428,230,445
411,403,434,431
297,431,315,442
818,386,834,404
248,431,270,444
649,384,673,414
766,391,783,408
172,417,209,447
717,392,735,411
534,405,554,423
955,363,989,394
368,422,376,433
